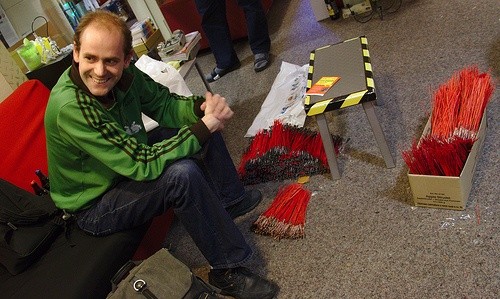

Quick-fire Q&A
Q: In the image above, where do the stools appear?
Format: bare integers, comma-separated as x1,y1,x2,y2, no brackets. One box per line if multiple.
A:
304,34,396,181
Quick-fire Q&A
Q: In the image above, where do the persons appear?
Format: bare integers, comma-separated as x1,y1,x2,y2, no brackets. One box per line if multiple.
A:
193,0,272,83
45,11,277,299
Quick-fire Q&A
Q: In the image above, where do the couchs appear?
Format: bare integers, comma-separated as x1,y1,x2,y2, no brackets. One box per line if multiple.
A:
0,79,175,299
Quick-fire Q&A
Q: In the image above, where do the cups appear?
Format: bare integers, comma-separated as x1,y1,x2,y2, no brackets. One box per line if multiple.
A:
15,42,45,72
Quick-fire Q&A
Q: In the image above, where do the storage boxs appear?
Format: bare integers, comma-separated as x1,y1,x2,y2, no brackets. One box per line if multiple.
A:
407,107,486,211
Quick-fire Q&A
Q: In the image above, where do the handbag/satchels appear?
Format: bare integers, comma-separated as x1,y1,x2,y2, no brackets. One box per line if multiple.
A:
0,194,64,277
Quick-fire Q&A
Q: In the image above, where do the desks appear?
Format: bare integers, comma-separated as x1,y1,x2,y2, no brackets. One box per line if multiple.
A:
177,58,214,101
25,47,73,93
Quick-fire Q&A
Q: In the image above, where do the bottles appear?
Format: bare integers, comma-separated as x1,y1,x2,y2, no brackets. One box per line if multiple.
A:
30,181,50,196
35,170,50,191
324,0,340,20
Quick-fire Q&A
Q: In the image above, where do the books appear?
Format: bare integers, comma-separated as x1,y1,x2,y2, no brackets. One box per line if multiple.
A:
304,76,342,96
126,18,203,60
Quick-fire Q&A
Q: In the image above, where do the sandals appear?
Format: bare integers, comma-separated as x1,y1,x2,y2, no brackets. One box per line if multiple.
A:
254,52,270,72
205,67,224,83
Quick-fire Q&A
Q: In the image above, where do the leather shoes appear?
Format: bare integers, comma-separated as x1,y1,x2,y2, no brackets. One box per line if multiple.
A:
226,188,262,220
208,266,278,298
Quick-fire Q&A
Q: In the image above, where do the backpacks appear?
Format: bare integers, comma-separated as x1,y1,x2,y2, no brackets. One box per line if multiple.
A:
106,247,217,298
